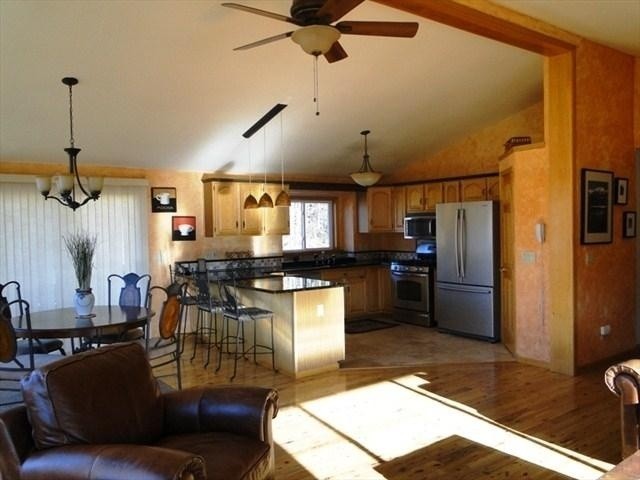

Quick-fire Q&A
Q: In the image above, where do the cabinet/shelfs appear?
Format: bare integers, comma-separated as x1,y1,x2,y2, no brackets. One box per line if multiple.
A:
296,270,391,322
357,176,498,236
498,142,552,369
203,182,289,237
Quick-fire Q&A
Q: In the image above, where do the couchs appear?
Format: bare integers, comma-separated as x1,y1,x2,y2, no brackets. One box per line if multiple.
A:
603,358,639,465
0,342,279,480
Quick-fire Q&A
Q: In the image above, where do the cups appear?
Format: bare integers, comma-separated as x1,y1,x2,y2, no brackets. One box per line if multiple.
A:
176,223,196,236
155,192,171,205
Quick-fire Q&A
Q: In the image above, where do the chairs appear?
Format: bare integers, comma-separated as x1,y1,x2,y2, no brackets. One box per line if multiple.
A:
0,282,67,370
213,277,278,380
138,283,188,391
172,264,213,355
189,272,233,369
76,274,148,351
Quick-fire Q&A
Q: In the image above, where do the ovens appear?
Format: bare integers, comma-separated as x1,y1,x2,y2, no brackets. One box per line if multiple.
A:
391,271,430,314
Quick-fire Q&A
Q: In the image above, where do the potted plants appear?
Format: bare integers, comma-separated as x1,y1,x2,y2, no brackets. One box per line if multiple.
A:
64,233,97,321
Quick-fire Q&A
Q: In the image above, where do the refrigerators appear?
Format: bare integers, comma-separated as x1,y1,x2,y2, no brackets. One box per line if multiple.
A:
433,199,501,345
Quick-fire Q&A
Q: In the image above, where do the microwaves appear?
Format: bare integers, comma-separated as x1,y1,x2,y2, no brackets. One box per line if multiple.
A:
402,215,436,241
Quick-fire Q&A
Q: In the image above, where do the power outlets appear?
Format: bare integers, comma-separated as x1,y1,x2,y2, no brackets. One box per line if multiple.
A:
601,324,611,336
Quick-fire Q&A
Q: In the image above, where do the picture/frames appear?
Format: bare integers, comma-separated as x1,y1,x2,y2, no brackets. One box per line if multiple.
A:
581,169,613,244
622,212,639,238
173,217,196,240
151,187,176,212
616,177,630,204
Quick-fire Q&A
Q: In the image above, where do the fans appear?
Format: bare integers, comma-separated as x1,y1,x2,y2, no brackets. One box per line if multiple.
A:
219,0,419,114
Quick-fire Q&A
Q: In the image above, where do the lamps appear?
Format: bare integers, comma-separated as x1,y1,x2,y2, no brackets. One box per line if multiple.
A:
35,78,103,212
276,104,292,208
257,122,274,208
349,130,383,188
242,125,259,209
